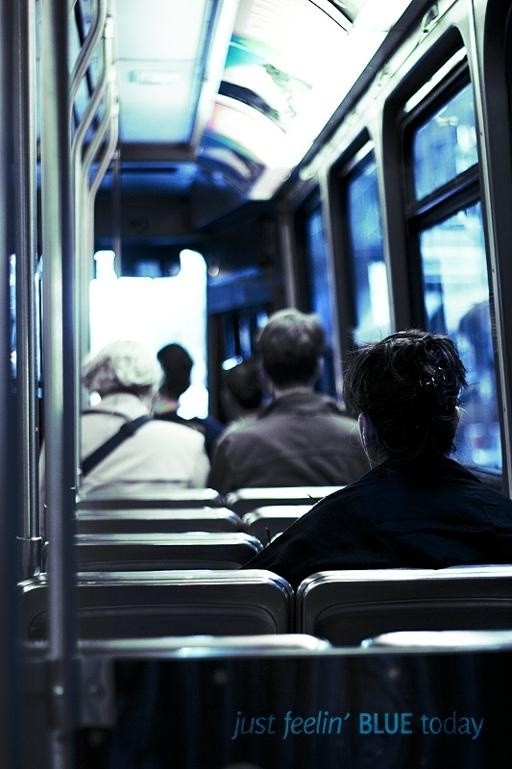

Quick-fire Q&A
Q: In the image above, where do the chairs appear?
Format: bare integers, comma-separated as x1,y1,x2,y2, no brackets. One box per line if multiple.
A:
15,481,511,769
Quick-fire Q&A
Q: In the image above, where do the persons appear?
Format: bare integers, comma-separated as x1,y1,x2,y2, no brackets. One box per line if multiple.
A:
149,344,224,456
208,306,371,499
212,357,266,452
235,328,512,595
79,342,211,496
